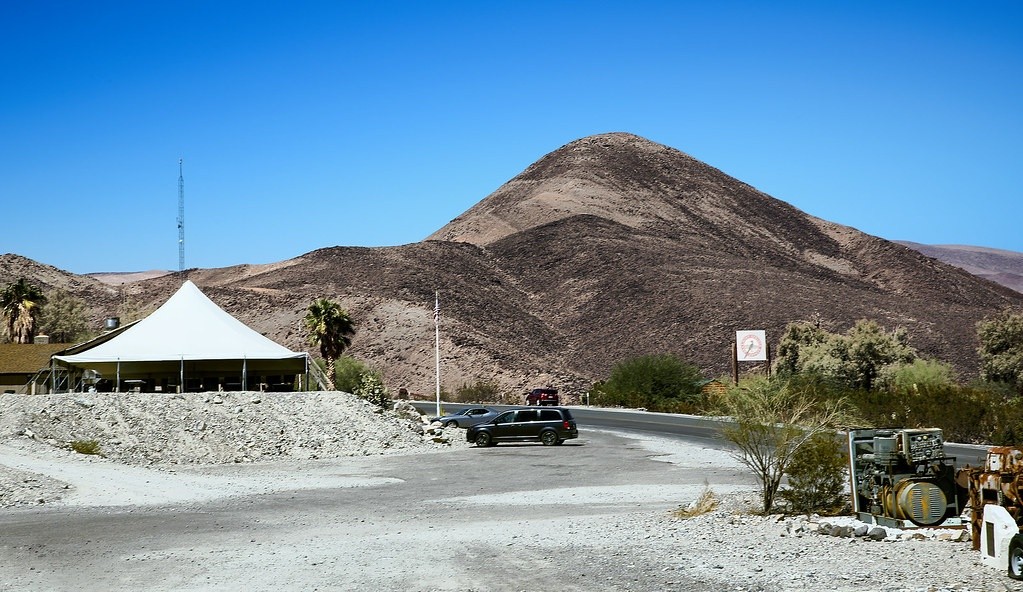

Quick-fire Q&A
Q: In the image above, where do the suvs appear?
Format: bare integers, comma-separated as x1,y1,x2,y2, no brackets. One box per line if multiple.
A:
466,406,579,448
521,386,558,406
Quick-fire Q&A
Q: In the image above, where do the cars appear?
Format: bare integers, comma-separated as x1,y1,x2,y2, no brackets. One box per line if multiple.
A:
437,405,504,428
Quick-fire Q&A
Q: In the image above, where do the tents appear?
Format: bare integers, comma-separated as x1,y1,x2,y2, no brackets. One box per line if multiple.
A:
15,278,338,392
